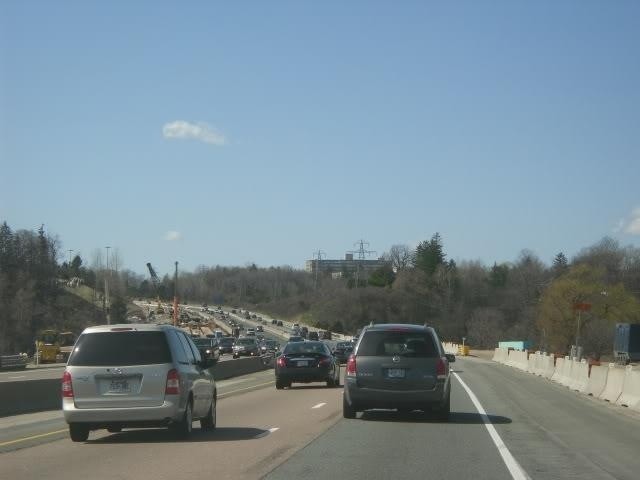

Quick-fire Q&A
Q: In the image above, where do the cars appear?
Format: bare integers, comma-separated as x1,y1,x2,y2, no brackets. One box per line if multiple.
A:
290,324,331,342
189,304,282,364
276,341,340,388
333,342,357,366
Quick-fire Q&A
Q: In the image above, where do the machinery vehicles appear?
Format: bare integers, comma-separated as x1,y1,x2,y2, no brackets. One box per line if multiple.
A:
36,328,60,362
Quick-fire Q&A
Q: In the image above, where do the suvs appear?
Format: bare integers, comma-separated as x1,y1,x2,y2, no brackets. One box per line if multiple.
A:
62,324,216,442
343,321,455,418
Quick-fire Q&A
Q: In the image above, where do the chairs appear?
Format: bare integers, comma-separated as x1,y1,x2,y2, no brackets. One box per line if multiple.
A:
404,337,425,357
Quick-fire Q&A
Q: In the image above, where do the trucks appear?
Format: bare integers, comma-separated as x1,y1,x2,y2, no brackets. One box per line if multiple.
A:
613,323,640,364
